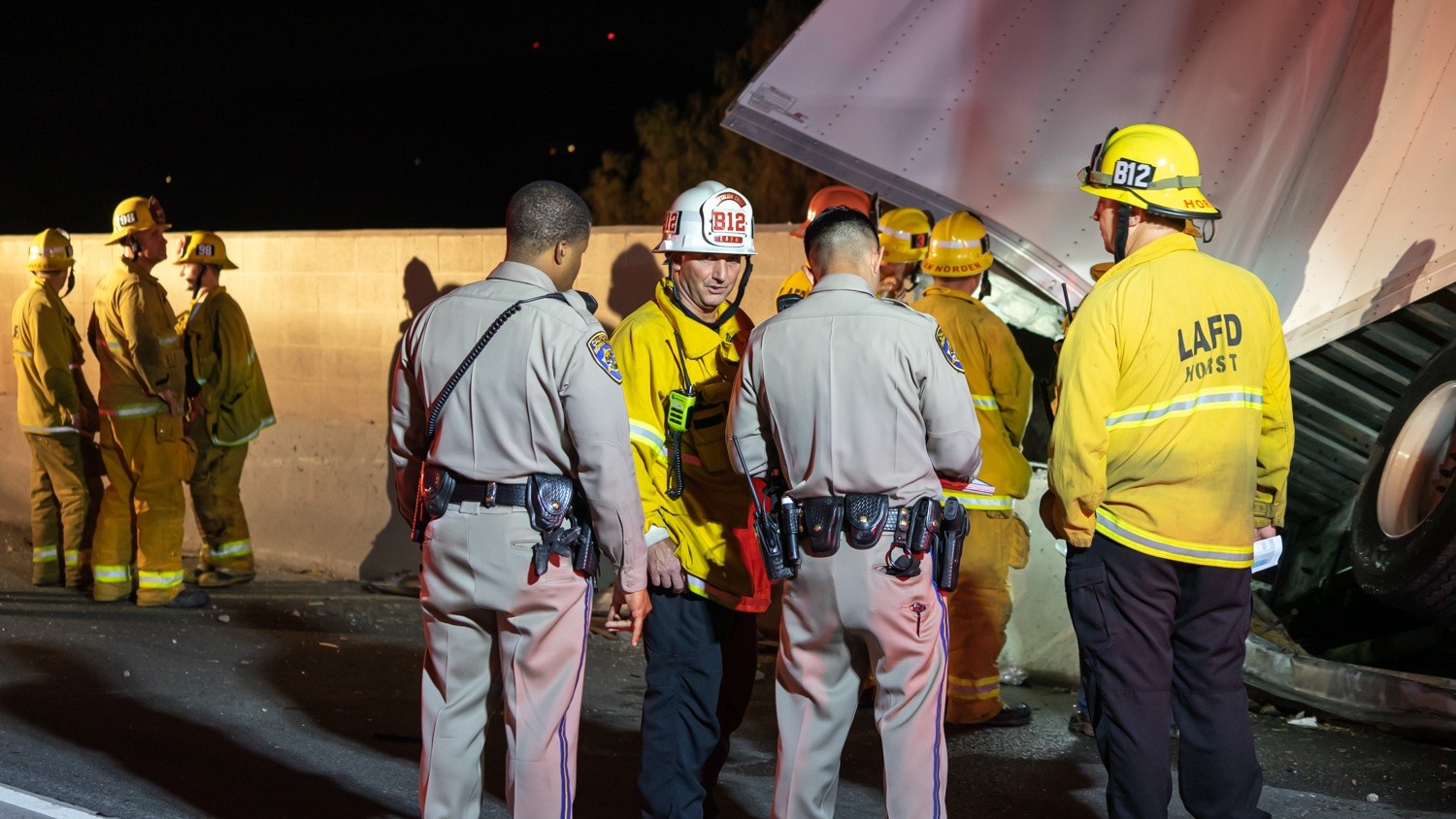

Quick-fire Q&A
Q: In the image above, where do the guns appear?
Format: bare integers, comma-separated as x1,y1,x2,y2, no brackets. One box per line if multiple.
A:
576,525,600,577
939,495,969,589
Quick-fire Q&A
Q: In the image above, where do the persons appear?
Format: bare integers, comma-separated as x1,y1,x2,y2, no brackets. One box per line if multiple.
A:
607,180,774,819
723,204,984,819
1038,124,1296,819
12,227,107,589
86,195,212,607
174,229,276,590
387,181,652,819
775,185,1032,730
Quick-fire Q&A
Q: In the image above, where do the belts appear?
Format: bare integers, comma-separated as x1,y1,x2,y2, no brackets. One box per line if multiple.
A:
449,481,527,507
838,508,911,532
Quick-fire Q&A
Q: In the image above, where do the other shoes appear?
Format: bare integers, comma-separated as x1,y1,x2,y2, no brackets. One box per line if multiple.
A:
140,585,210,610
1069,710,1094,736
858,689,874,708
69,582,94,590
183,568,216,583
1171,724,1180,738
948,702,1032,727
35,576,65,586
198,569,255,587
95,580,139,604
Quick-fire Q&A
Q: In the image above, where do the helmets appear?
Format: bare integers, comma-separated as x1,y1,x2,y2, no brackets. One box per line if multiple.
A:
788,185,871,238
1078,124,1222,220
27,227,75,271
877,208,932,262
651,180,759,255
1090,264,1116,282
172,231,239,270
103,196,172,246
921,210,993,277
